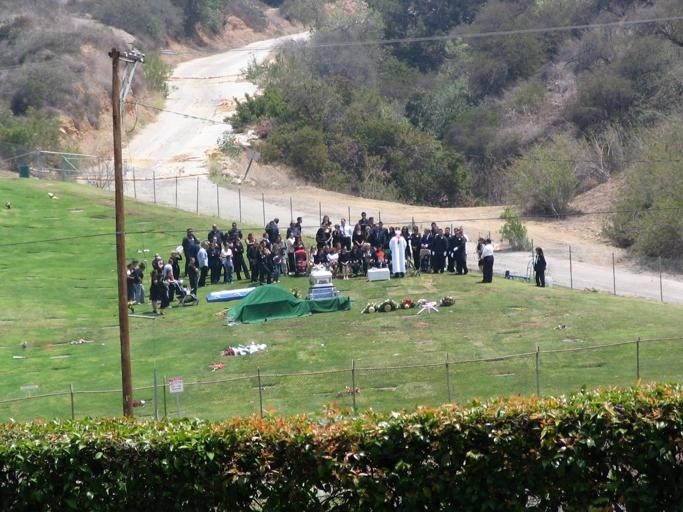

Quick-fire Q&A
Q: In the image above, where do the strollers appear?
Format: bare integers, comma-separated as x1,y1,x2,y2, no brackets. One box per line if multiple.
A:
170,278,199,307
293,249,309,277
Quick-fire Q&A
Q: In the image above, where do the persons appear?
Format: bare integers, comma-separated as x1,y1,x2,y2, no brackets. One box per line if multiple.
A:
534,247,546,288
475,238,494,283
182,211,469,297
125,249,182,316
475,237,488,271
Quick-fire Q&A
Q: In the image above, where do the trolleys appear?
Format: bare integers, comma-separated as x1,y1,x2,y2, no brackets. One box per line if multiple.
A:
504,259,534,282
